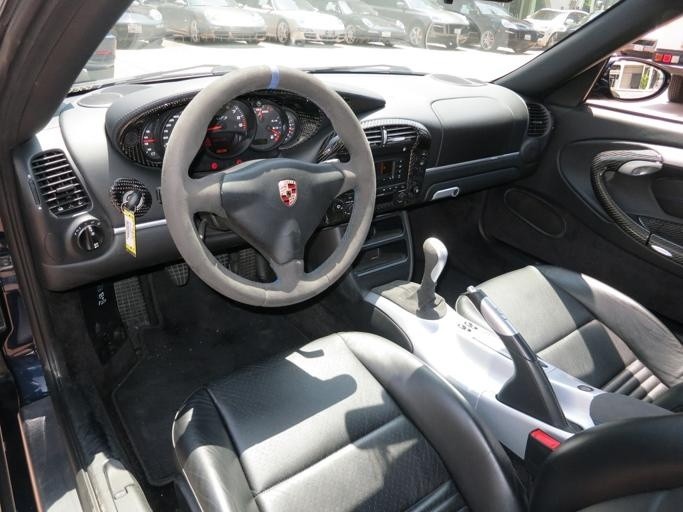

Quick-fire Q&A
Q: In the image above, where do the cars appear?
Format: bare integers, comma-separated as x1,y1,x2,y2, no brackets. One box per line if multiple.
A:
522,7,590,48
154,1,268,46
310,1,408,46
71,31,116,84
616,36,683,102
237,1,345,46
110,1,166,48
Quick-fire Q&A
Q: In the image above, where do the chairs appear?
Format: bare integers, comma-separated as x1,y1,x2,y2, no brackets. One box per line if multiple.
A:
456,260,682,413
173,327,682,508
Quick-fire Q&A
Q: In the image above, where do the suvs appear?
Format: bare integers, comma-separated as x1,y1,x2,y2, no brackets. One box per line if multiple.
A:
375,1,472,50
445,0,539,55
